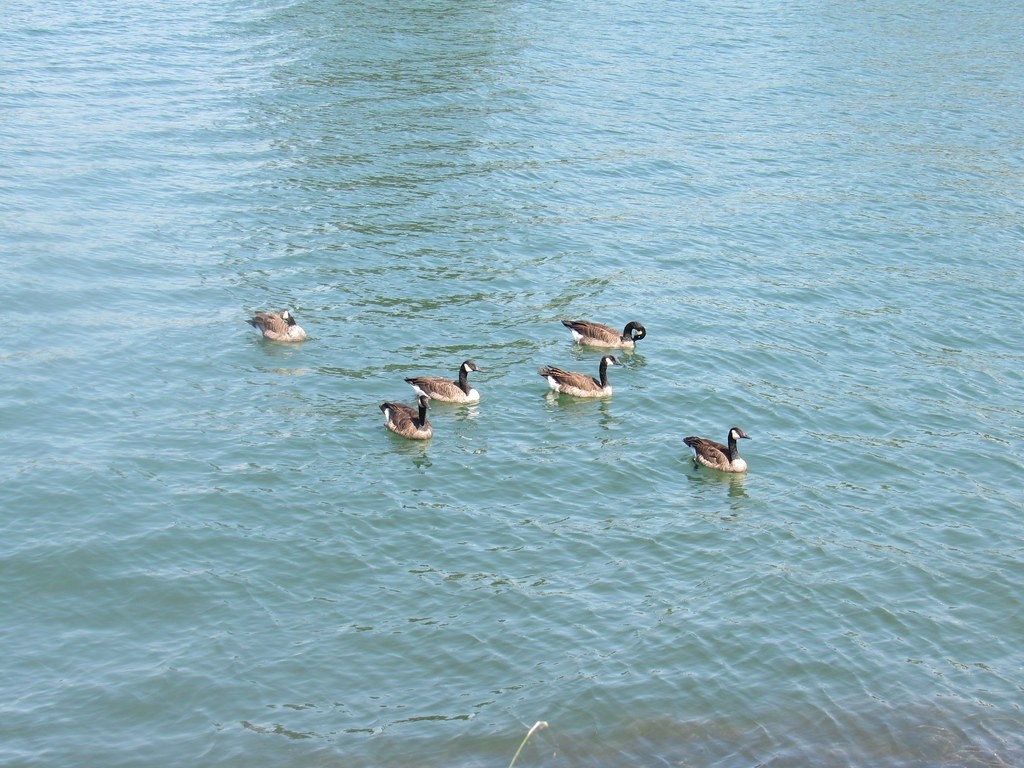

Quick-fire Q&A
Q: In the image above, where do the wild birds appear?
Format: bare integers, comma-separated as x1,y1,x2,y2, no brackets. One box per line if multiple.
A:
683,426,752,473
561,319,647,348
538,354,622,397
379,395,433,440
245,309,306,342
404,360,482,404
